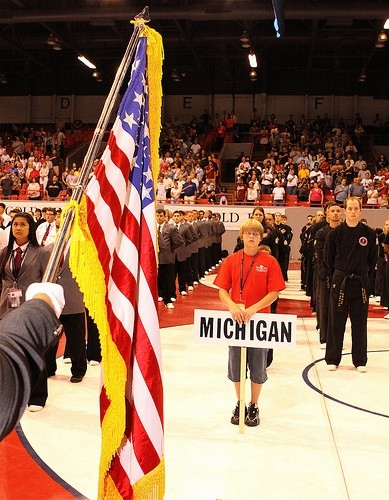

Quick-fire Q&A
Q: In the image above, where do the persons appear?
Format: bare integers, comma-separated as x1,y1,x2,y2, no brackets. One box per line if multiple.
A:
218,219,280,426
0,201,102,384
0,282,65,442
0,114,94,201
323,196,377,373
0,212,52,413
158,108,389,348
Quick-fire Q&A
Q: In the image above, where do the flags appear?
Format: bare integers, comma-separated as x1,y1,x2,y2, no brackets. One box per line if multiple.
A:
69,15,167,500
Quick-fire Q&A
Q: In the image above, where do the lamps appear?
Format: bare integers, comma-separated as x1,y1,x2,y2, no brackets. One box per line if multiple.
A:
358,69,367,82
375,15,389,48
46,32,62,50
240,30,258,81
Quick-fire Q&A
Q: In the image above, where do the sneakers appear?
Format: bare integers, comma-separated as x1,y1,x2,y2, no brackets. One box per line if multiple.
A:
231,400,247,425
245,403,260,427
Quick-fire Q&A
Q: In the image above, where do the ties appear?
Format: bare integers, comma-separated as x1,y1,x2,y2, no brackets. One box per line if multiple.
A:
40,223,51,247
12,247,23,278
175,224,178,229
158,225,161,233
